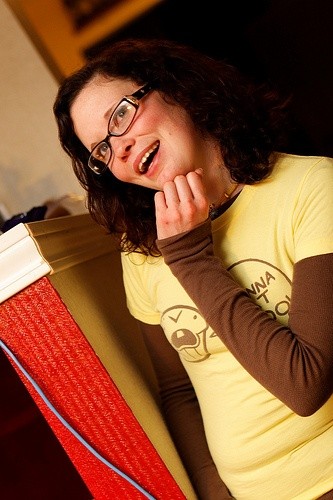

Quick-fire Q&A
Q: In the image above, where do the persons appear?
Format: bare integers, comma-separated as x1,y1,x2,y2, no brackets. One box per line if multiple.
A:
51,44,333,500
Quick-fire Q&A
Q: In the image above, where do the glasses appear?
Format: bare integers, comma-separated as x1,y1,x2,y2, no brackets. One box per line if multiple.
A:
86,83,155,176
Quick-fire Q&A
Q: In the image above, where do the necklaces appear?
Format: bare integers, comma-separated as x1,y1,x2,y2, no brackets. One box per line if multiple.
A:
205,178,239,217
